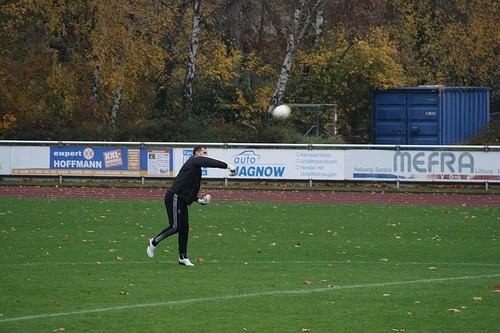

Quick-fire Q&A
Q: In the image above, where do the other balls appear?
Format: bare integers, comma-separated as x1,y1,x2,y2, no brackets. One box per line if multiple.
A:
271,105,291,120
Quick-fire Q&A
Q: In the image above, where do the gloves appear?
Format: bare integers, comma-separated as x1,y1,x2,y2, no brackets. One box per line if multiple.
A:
198,197,210,206
228,165,237,176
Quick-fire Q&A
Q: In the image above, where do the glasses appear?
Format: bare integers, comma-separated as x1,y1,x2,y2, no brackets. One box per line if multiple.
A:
200,151,206,154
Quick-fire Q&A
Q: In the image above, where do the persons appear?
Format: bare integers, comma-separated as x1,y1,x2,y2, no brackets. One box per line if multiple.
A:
145,145,236,267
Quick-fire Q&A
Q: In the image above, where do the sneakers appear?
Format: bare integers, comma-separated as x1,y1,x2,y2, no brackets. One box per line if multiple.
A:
147,238,156,257
178,257,194,266
